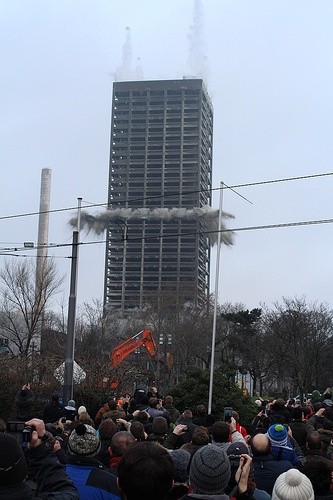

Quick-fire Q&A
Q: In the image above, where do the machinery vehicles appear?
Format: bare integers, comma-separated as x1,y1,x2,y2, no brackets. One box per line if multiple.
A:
73,329,175,392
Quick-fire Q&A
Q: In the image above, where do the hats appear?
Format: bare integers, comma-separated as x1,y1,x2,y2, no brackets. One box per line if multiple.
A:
68,423,101,458
0,433,27,484
227,443,247,456
190,445,231,493
78,406,86,416
255,399,261,407
169,449,191,482
152,416,167,432
272,468,314,500
267,424,287,445
68,400,75,406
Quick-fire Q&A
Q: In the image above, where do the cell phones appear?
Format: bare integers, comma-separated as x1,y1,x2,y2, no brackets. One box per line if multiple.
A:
223,407,233,424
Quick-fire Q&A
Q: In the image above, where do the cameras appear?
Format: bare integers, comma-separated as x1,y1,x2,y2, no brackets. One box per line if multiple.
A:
6,421,37,442
228,454,242,468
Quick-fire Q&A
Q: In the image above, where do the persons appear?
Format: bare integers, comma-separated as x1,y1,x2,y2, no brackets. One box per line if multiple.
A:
0,383,333,500
